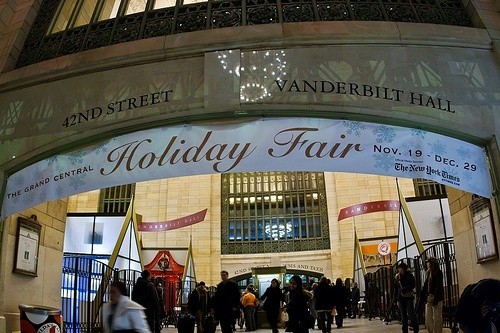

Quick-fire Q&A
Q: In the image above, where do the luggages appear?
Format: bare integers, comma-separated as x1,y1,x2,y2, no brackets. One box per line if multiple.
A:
177,313,195,333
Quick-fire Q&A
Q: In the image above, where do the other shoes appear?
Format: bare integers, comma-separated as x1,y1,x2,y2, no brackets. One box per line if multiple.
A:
351,315,356,319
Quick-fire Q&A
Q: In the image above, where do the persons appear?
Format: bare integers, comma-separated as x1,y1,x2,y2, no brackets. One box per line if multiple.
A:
327,278,362,320
332,278,347,328
216,270,242,333
240,284,259,332
282,275,313,333
102,281,151,333
363,282,380,315
257,279,283,333
418,256,444,333
190,280,216,333
307,282,318,326
315,276,335,333
394,263,419,333
130,270,167,333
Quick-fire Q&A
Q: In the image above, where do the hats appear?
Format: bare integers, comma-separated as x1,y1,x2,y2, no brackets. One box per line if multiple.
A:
425,258,438,263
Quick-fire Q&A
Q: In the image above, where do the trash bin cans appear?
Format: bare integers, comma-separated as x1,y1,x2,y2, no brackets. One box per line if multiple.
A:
18,304,61,333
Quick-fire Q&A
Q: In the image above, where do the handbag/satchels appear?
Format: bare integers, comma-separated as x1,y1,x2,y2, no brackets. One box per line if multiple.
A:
400,289,413,297
